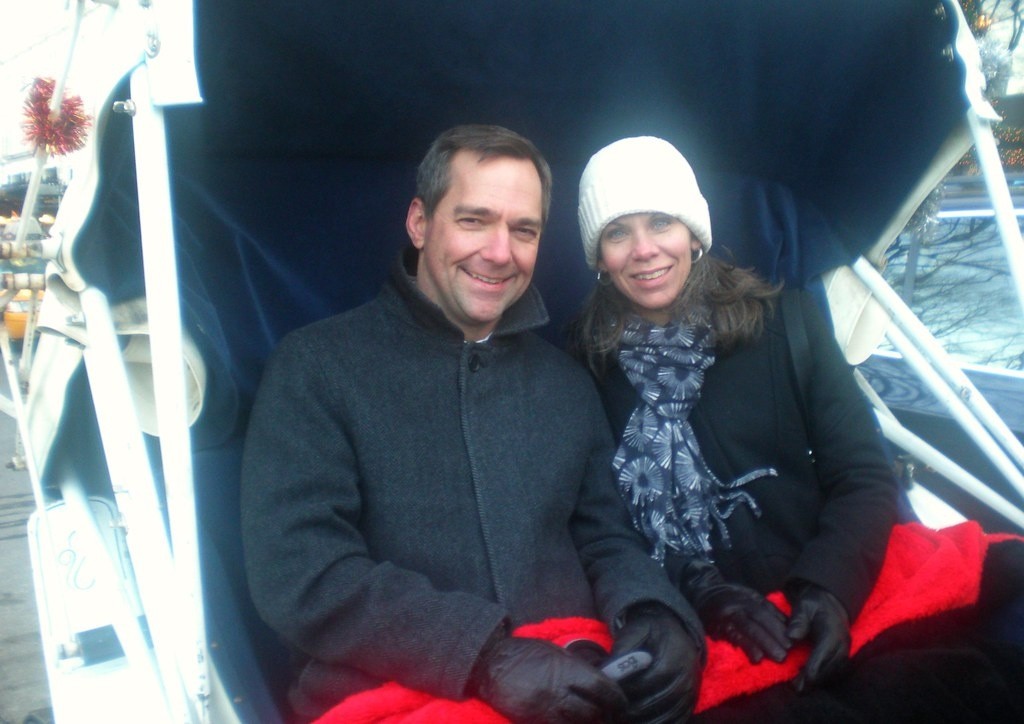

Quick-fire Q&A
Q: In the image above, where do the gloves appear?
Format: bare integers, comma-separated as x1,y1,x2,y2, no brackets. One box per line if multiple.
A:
479,637,627,724
684,568,796,667
786,584,853,695
609,608,702,723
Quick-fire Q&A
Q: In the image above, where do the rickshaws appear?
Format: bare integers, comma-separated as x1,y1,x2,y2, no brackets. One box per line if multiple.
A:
0,1,1024,724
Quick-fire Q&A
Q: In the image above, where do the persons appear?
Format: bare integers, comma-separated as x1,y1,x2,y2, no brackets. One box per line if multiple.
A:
239,125,709,724
561,137,1024,724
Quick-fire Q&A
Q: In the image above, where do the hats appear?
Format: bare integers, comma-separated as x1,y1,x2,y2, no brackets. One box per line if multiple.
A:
578,135,712,272
